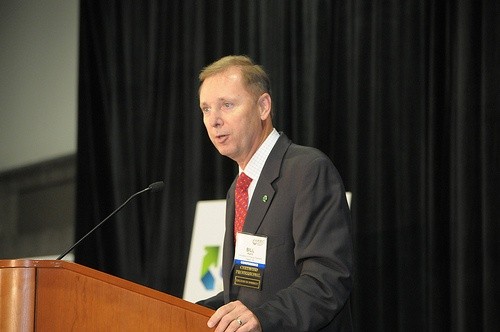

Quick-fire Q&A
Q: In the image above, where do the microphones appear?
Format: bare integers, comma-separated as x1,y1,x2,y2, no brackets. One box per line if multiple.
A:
57,181,166,259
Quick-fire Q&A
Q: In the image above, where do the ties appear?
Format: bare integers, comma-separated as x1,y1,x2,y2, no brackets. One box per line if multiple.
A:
232,172,253,247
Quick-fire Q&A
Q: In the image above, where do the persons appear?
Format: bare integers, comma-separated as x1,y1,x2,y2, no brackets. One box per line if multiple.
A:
199,56,360,332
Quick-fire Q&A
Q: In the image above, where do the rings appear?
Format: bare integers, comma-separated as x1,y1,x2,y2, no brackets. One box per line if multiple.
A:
236,319,242,327
